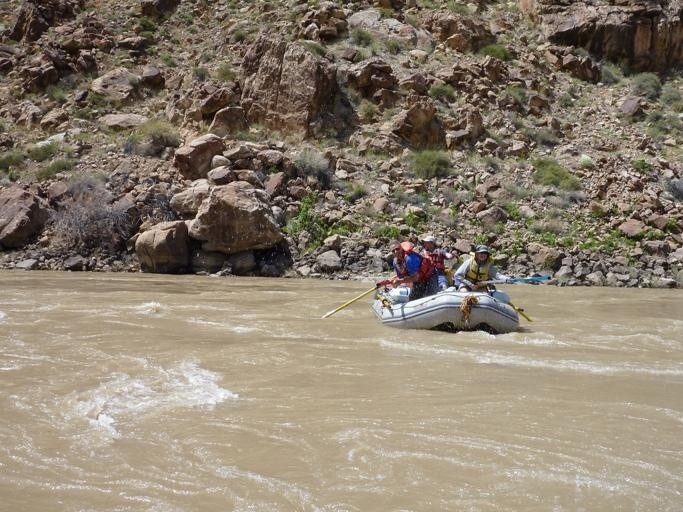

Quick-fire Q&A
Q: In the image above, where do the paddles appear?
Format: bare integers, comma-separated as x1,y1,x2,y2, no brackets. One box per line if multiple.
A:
321,276,397,319
476,276,550,285
373,290,393,310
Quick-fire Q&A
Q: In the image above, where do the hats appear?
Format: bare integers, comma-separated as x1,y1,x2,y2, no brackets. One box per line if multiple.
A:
422,236,436,243
476,246,492,255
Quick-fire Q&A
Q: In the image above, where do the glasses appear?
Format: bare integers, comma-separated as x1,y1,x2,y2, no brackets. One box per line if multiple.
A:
392,248,399,252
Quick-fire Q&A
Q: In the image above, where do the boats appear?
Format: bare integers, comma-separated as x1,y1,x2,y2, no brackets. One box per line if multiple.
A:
370,281,520,336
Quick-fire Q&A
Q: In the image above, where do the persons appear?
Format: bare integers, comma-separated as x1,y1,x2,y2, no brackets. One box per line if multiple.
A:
452,244,515,298
421,235,457,291
387,239,438,300
